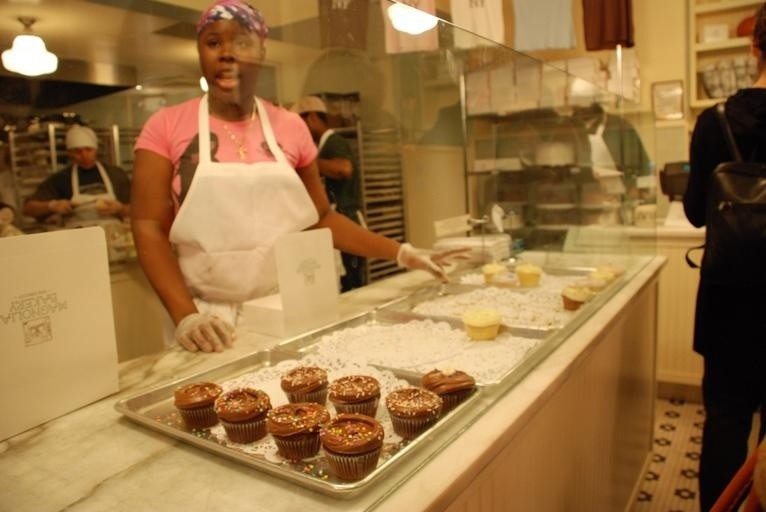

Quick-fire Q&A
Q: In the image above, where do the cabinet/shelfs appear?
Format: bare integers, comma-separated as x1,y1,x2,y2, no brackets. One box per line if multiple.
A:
0,248,670,512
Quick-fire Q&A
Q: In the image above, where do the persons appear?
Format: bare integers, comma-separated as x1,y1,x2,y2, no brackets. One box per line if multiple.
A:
423,50,651,251
131,0,472,352
23,126,131,230
682,4,766,512
290,96,369,294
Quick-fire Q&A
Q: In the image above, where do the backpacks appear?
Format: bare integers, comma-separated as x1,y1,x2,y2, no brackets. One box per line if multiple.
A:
705,102,766,289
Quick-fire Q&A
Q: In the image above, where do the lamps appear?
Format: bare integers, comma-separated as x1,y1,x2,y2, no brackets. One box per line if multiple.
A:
0,16,58,75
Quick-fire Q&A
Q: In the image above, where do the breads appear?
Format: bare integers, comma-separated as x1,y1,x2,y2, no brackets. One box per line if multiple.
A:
561,264,623,310
481,263,505,283
461,306,501,341
514,263,542,288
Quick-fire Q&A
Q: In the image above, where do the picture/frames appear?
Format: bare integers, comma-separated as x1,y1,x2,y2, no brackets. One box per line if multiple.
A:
651,79,685,120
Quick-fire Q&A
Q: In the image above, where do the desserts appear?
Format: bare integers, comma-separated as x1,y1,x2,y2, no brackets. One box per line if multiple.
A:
421,367,476,415
319,412,384,483
281,365,329,407
329,375,381,420
386,387,443,440
264,401,331,461
213,386,272,444
174,382,222,430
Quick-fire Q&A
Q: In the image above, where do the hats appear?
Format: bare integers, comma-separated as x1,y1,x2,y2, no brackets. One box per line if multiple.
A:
568,79,593,108
196,0,267,38
297,96,327,117
66,125,98,150
